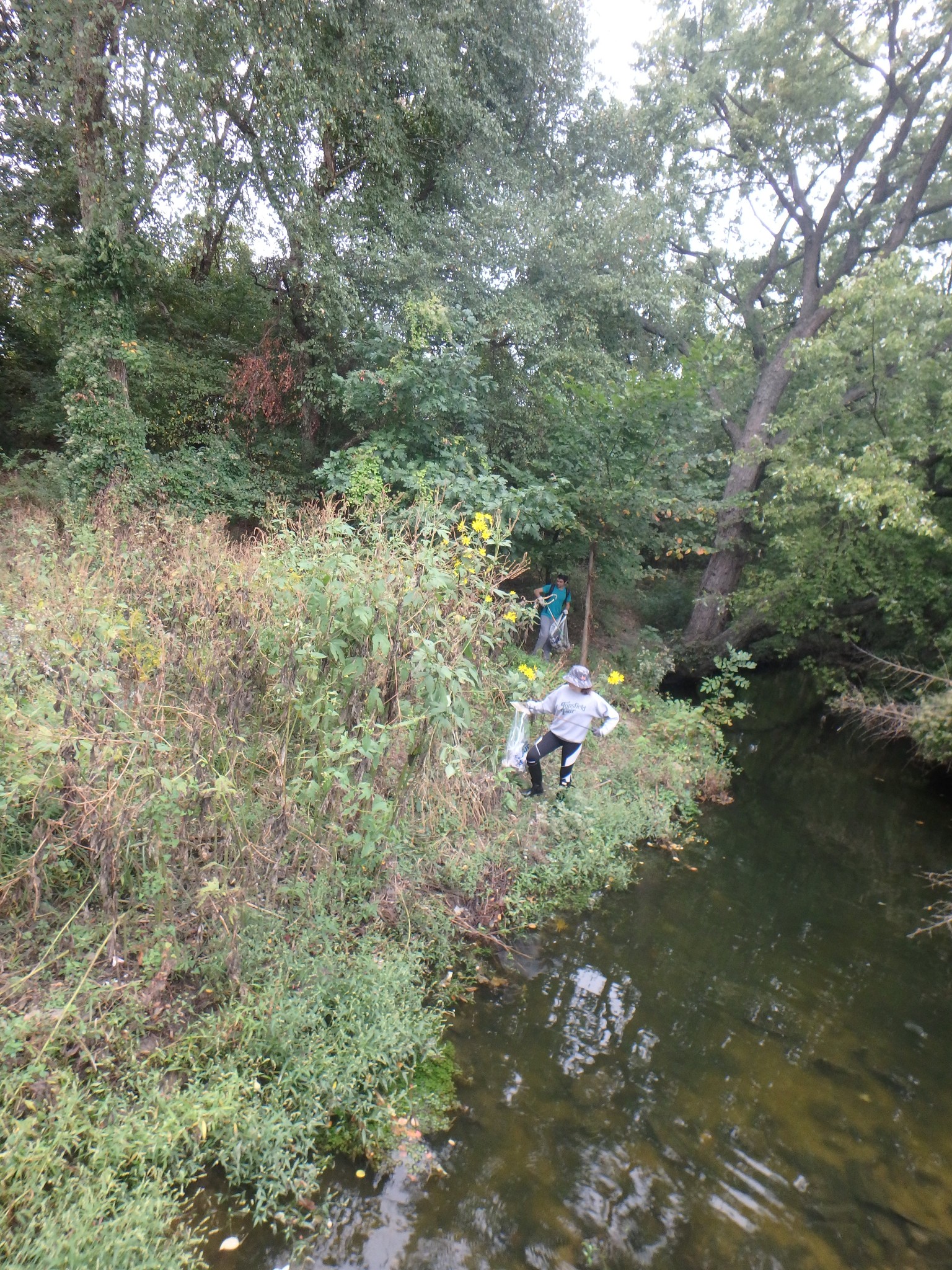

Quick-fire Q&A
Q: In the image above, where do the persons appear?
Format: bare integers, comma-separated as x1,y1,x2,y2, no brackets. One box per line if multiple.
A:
521,665,620,802
532,574,571,661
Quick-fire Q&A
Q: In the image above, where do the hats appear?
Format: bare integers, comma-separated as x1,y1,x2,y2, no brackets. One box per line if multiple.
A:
562,665,592,689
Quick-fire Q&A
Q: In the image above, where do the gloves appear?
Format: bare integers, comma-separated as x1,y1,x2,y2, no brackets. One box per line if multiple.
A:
520,702,528,708
591,726,601,737
562,609,569,615
538,596,546,608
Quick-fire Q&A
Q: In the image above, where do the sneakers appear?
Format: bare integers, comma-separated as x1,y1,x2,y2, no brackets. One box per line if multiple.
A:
520,786,543,798
